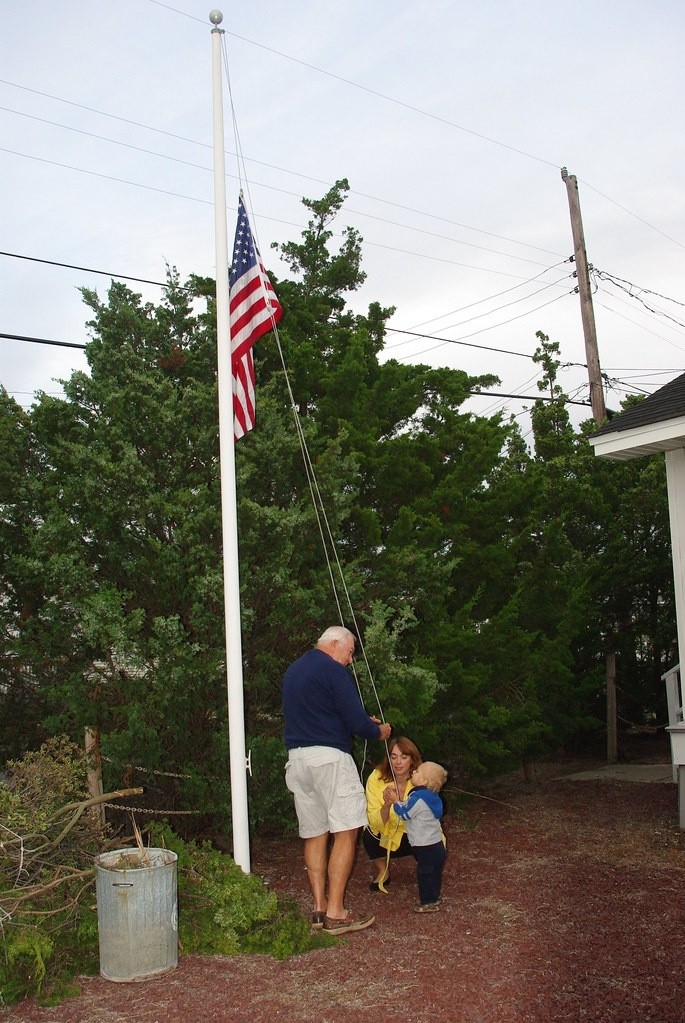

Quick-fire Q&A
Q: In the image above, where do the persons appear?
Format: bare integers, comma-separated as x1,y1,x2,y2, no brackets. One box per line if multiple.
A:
363,735,446,890
281,626,391,936
388,760,449,911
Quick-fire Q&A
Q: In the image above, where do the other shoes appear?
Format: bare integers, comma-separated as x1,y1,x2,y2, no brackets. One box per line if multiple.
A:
414,898,442,912
370,876,390,891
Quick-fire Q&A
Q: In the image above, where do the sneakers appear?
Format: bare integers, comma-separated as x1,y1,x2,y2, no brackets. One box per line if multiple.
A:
321,909,375,935
309,911,327,928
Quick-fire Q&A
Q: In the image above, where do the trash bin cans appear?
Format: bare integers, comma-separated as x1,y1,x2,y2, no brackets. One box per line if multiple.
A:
93,847,181,983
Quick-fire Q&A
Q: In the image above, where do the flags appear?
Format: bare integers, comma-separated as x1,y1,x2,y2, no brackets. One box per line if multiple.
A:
229,190,283,441
